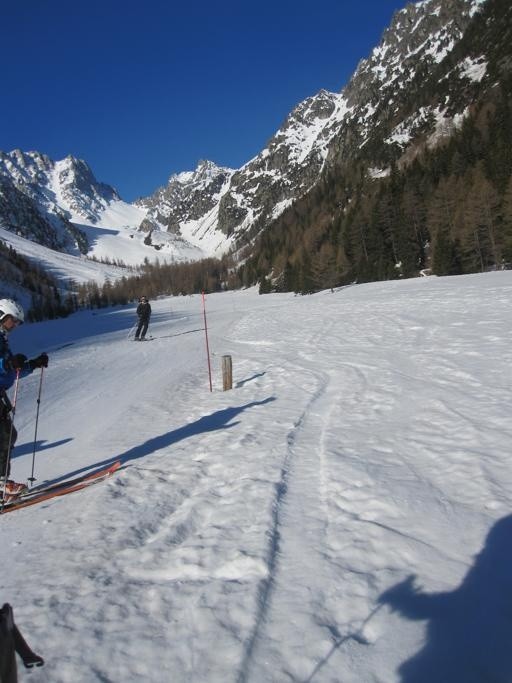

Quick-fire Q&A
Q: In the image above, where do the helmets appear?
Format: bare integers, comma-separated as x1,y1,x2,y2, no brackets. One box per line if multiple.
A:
0,298,24,322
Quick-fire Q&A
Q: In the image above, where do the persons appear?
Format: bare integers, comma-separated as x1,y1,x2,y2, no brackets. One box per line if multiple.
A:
134,295,151,343
0,297,49,494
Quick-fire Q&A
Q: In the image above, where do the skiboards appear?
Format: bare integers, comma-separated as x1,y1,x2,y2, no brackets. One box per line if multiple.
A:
1,460,121,513
131,337,155,342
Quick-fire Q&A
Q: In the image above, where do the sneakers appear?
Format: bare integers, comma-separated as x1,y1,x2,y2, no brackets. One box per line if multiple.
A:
0,480,28,507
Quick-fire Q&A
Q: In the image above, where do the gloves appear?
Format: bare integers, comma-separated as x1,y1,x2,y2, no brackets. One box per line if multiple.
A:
29,354,48,368
6,354,28,368
